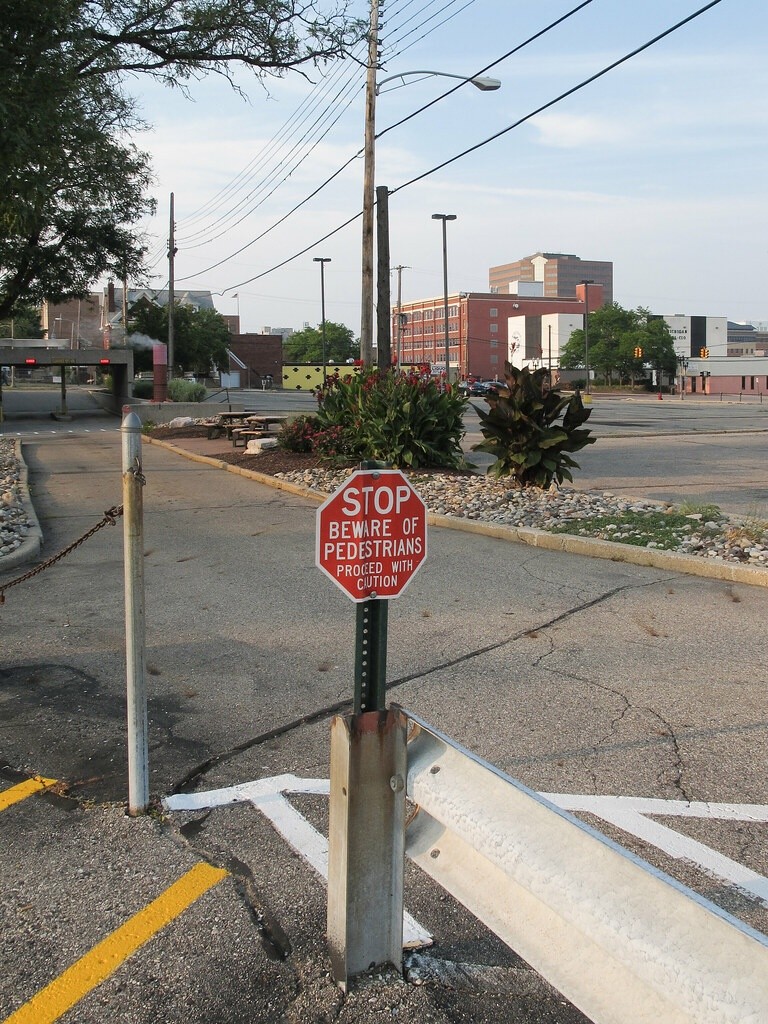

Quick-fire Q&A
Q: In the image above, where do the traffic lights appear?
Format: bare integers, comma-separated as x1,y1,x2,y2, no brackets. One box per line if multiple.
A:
700,348,705,358
639,347,643,358
707,372,711,377
705,349,710,359
700,371,704,377
634,347,638,358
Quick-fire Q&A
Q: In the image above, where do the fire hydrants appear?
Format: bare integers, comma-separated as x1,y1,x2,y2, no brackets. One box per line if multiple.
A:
657,391,663,400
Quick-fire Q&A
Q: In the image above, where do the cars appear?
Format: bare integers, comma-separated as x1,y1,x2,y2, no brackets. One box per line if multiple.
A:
481,382,510,395
457,381,487,397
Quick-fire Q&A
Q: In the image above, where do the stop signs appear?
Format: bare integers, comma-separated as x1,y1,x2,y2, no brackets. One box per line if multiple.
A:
313,469,429,605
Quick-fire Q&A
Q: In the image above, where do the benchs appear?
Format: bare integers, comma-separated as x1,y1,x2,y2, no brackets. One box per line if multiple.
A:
232,428,270,447
239,430,284,449
201,422,233,440
222,424,264,440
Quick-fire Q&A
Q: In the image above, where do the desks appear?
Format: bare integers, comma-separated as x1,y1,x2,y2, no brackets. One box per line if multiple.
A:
218,411,258,438
246,416,289,442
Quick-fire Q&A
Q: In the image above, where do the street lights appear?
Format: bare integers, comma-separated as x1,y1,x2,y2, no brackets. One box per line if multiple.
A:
431,214,457,398
55,317,75,349
579,279,595,396
313,257,331,389
